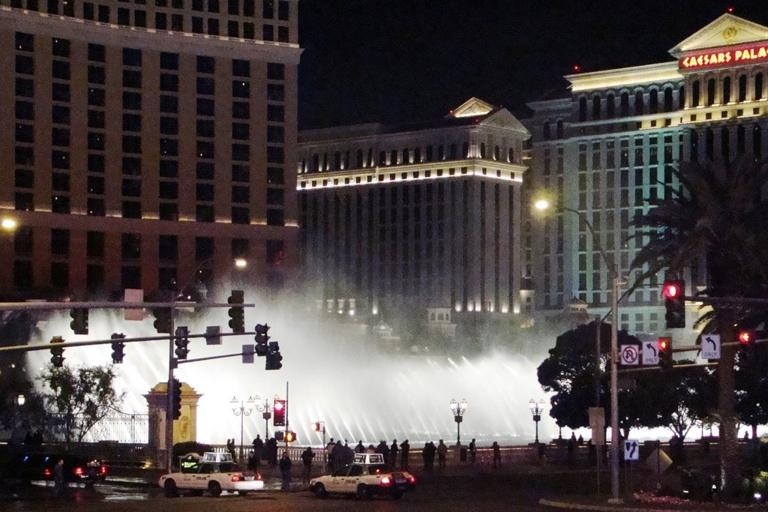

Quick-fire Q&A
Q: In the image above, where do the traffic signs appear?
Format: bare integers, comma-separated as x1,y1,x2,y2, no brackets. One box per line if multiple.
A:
700,334,720,360
641,341,658,365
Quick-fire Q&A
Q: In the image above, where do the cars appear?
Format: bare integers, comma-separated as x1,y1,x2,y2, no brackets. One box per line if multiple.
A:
349,451,383,465
309,463,416,501
158,460,264,497
9,447,111,487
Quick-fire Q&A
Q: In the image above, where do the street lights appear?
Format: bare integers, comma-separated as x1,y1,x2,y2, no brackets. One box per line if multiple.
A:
532,196,620,507
255,394,280,445
69,296,88,335
449,397,467,447
230,396,254,460
528,398,546,444
50,335,66,365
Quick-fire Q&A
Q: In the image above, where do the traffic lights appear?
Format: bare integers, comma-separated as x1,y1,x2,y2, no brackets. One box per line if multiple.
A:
658,336,672,368
226,290,246,335
285,430,296,443
175,326,190,359
739,330,757,369
153,298,171,333
265,341,282,370
110,334,126,364
254,324,270,356
273,400,285,426
663,279,686,329
167,379,181,420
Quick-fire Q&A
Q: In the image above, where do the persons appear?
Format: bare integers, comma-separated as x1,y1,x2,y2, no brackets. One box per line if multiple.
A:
677,447,691,502
227,433,278,473
324,437,502,478
743,430,749,441
567,429,598,470
300,446,316,488
702,440,712,459
50,457,68,497
669,435,682,466
279,451,292,491
